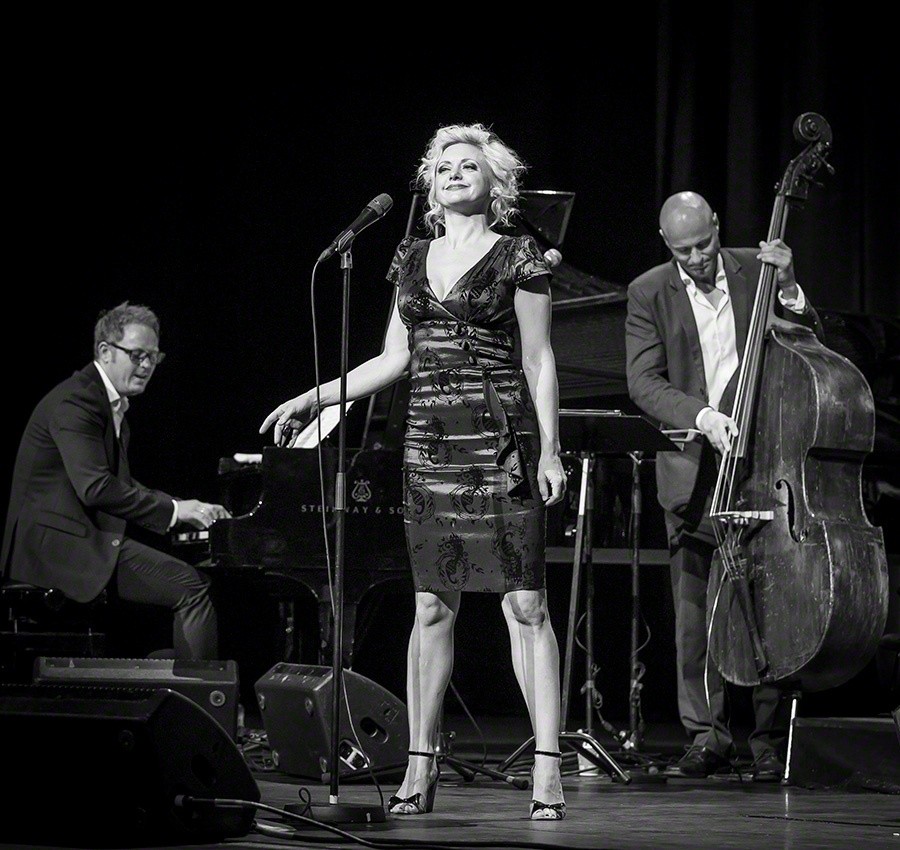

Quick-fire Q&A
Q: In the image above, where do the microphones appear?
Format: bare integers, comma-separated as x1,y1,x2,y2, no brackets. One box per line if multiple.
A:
542,249,562,268
319,193,394,263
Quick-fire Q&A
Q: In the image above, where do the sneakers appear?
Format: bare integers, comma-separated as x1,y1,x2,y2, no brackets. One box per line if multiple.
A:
754,748,787,781
679,745,732,777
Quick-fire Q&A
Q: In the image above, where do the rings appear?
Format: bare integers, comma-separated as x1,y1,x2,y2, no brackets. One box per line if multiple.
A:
201,507,204,514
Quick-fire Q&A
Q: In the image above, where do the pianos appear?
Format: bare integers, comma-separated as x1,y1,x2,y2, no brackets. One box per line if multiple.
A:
168,188,669,667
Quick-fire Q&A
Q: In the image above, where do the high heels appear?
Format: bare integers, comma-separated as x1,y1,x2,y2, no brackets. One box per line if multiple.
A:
388,751,438,814
529,750,566,822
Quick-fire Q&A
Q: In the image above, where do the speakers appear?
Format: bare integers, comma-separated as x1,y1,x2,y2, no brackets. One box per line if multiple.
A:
0,655,260,850
254,660,409,780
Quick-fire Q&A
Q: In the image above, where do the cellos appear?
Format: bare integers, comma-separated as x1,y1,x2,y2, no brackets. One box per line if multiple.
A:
705,112,891,786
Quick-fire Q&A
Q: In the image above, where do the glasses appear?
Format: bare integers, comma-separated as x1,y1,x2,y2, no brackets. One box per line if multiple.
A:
107,342,165,364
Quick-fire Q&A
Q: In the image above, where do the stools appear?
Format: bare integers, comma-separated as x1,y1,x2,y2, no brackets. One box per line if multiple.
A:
0,582,110,635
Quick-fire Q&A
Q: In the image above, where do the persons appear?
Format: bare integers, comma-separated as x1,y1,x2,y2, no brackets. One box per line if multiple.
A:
0,300,233,660
625,191,822,783
260,123,567,819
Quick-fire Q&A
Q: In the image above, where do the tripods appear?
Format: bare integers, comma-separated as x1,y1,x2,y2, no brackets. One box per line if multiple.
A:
322,231,686,790
